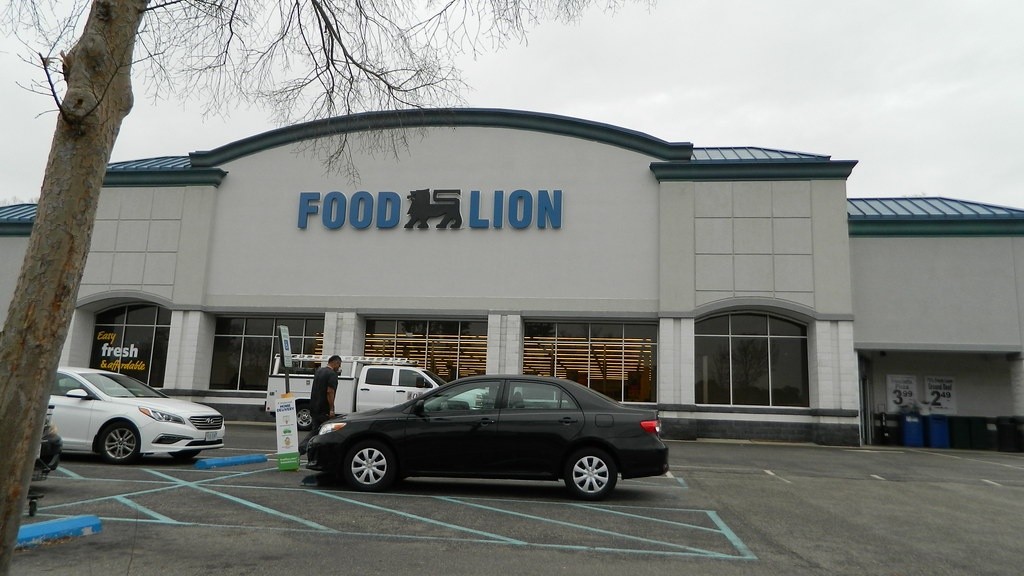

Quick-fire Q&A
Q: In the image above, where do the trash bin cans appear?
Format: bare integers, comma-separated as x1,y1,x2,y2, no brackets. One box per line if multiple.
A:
873,412,1024,452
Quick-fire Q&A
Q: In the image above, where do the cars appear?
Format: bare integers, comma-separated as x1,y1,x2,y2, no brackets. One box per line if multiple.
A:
42,363,226,465
304,371,672,503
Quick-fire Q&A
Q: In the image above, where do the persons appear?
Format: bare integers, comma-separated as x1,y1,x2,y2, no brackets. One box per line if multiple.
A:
297,354,342,456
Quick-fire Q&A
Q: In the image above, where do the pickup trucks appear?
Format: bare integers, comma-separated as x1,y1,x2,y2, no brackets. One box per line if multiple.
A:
265,354,488,432
